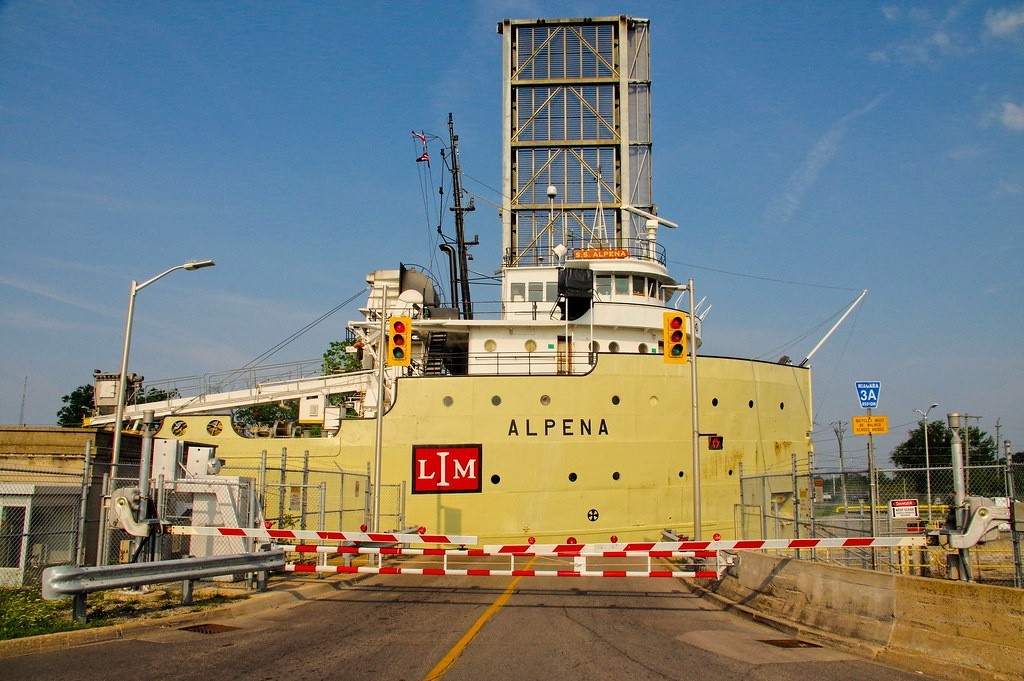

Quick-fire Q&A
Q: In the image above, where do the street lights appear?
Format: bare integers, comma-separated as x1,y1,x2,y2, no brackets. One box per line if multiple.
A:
103,260,217,562
660,278,701,540
910,403,938,524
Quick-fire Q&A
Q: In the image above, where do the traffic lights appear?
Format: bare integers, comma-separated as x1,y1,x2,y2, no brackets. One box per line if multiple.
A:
662,312,687,365
387,316,411,367
